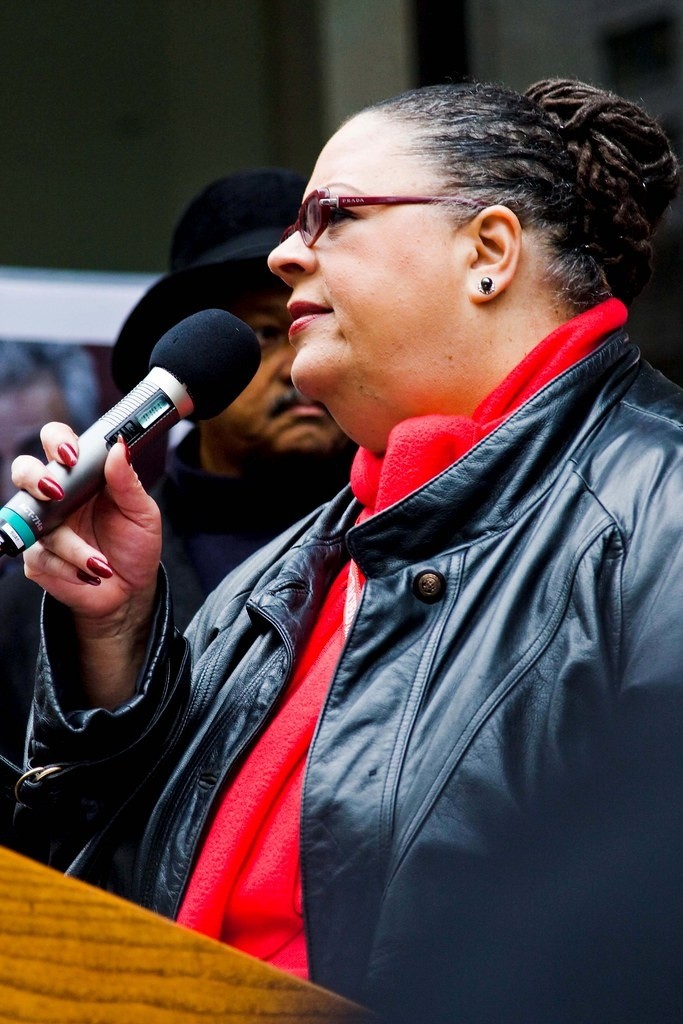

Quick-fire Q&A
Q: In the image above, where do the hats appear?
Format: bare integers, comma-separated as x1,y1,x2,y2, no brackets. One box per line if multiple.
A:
110,170,309,397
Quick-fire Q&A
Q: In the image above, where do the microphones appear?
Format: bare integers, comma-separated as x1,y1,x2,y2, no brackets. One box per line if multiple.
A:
0,307,263,556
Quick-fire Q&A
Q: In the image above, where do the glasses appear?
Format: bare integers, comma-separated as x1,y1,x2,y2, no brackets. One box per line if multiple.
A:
281,186,527,247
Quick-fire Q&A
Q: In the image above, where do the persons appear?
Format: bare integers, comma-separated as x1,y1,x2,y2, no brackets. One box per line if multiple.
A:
0,168,362,776
0,80,683,1024
